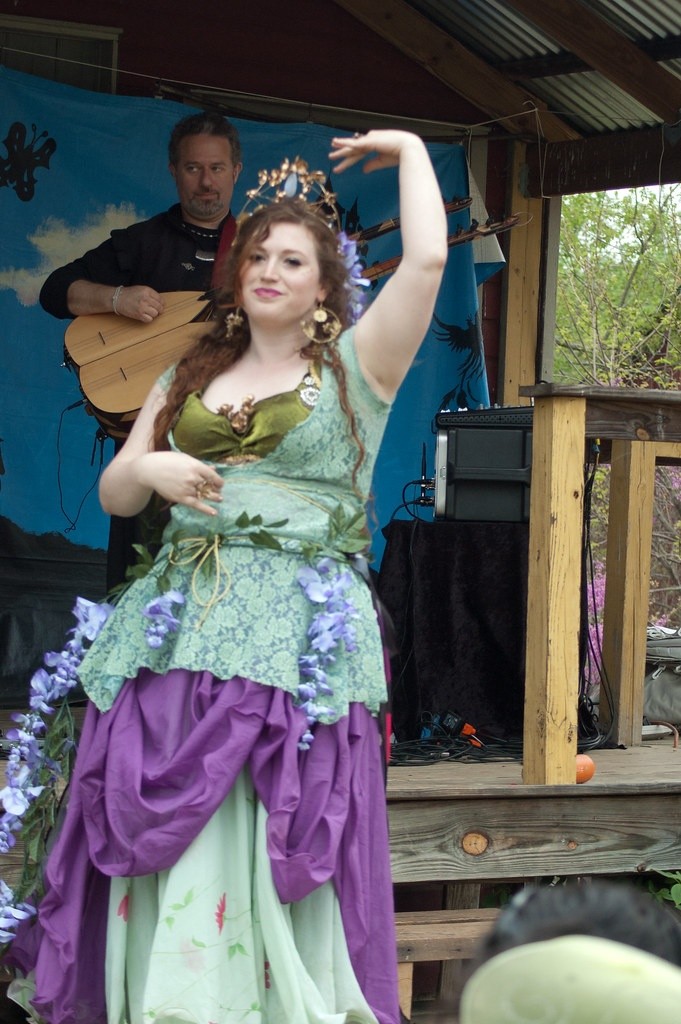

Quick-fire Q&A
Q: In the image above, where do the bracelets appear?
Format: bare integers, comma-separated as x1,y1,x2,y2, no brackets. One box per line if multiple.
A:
112,286,124,316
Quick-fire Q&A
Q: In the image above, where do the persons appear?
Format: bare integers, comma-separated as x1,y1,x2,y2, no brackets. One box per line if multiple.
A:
445,877,681,1024
39,111,251,603
3,128,450,1024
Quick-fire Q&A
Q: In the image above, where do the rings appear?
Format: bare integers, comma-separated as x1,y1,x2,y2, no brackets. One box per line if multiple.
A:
193,480,213,500
353,132,363,139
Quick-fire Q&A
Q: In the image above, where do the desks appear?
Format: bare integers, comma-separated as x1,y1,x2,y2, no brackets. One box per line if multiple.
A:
377,518,530,748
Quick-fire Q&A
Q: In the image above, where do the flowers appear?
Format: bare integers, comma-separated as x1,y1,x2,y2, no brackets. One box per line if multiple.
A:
337,231,370,326
0,500,373,975
